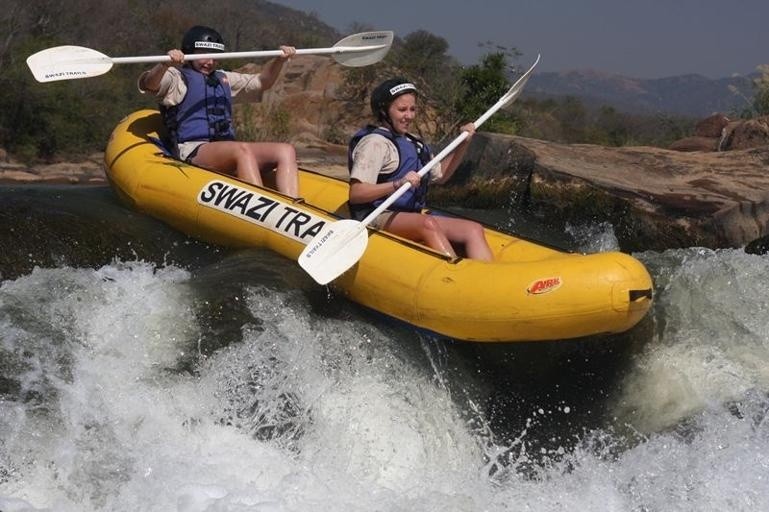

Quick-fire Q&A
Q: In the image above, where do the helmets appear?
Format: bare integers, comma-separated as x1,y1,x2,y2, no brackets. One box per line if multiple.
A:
181,25,225,52
370,77,419,111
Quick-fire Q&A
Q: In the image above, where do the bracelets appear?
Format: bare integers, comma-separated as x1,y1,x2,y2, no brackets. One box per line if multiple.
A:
393,179,404,190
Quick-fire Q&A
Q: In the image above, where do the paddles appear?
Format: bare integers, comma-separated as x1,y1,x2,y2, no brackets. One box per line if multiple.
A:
297,53,542,285
26,30,395,83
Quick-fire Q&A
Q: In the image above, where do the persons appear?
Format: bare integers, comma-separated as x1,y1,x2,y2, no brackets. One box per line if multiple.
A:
348,77,496,263
137,26,298,199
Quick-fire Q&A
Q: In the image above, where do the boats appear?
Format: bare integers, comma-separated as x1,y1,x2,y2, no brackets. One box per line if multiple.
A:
104,109,654,341
